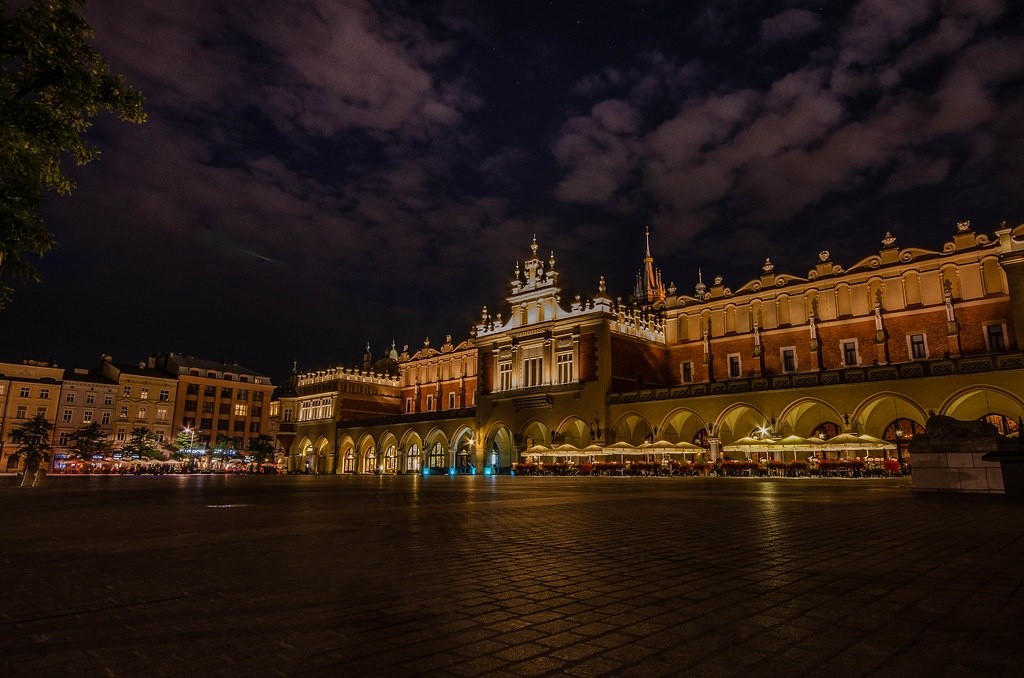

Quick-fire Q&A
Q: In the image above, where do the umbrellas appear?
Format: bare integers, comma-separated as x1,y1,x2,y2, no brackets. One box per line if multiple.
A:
61,458,184,469
723,433,897,477
521,441,706,476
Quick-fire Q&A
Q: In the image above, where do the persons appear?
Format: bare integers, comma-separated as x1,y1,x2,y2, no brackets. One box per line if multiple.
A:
468,462,473,473
715,458,723,478
233,468,248,476
515,458,700,477
760,456,768,477
112,466,116,473
129,464,135,473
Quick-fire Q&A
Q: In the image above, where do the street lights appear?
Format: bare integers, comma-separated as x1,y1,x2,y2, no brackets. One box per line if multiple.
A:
185,428,194,457
